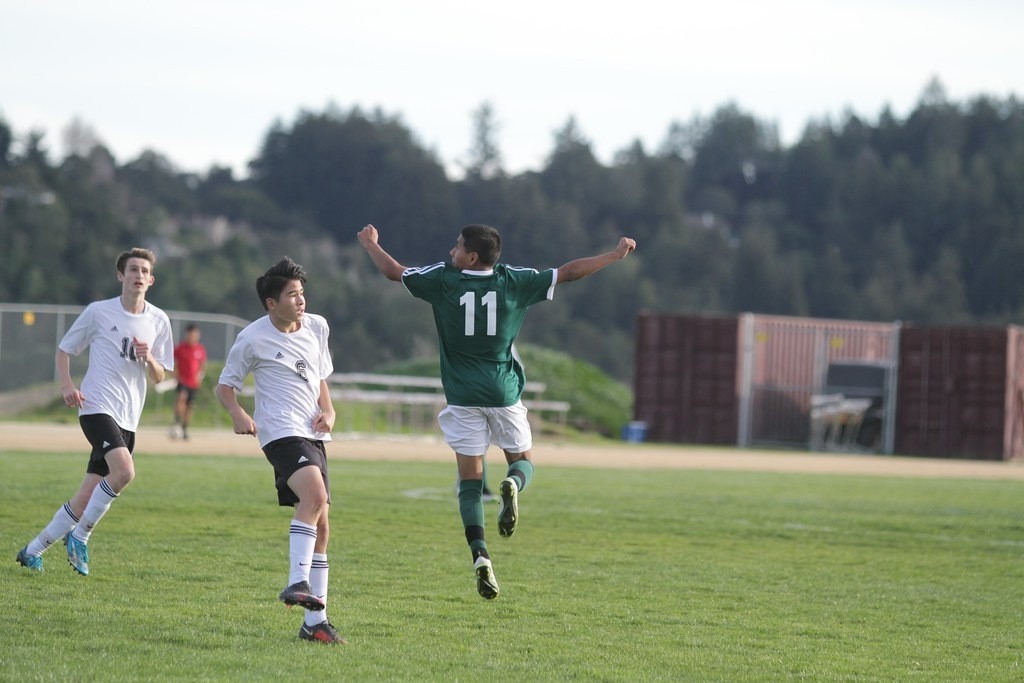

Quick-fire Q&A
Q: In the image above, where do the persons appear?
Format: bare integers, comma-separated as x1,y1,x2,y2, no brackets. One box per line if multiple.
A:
17,248,175,576
357,223,637,598
171,323,207,440
214,259,347,647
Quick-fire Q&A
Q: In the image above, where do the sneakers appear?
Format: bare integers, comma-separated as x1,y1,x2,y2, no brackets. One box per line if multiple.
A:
16,545,44,573
473,551,499,600
279,580,325,611
497,478,518,539
62,527,88,576
298,619,349,644
